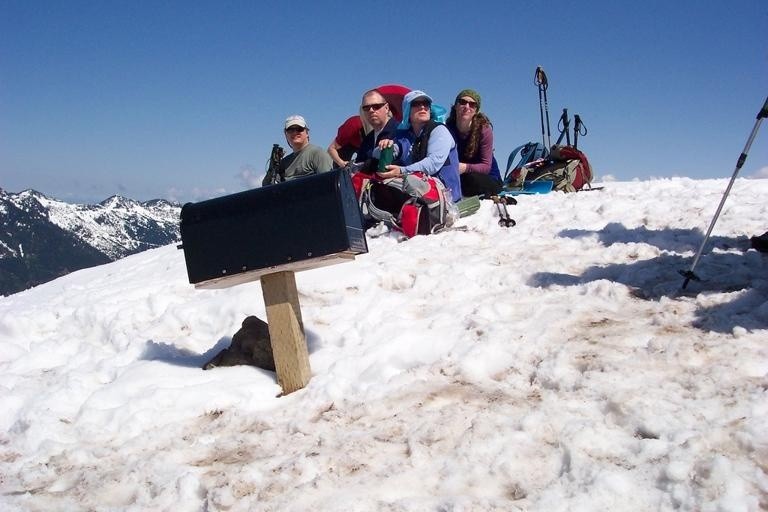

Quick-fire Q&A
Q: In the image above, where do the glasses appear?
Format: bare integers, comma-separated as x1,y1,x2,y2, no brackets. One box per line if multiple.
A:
412,100,431,107
362,103,386,111
287,127,305,133
457,98,476,108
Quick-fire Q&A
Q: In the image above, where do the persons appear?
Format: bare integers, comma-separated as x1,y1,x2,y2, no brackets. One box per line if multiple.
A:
328,89,462,215
446,90,503,199
262,115,333,187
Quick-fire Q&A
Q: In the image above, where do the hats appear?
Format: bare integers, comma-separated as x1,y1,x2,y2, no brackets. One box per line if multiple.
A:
284,115,309,130
402,90,432,126
455,89,481,112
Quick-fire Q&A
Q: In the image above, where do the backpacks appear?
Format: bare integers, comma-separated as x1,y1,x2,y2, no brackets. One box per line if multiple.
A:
358,172,458,241
504,142,593,193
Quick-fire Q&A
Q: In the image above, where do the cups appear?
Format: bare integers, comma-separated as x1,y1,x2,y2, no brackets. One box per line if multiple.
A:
377,142,396,172
